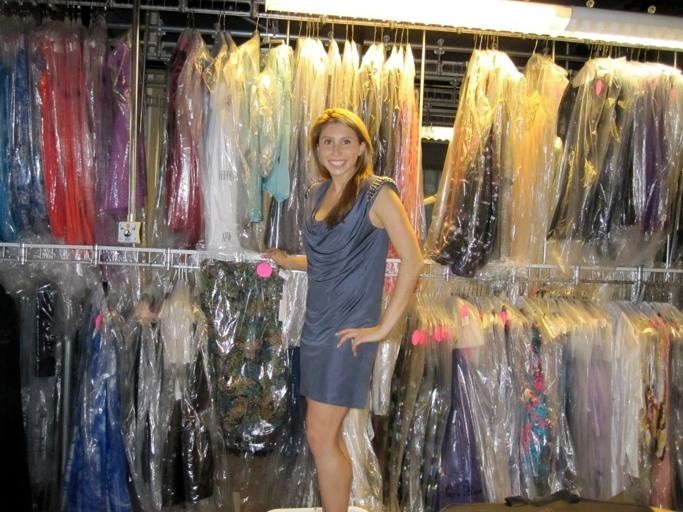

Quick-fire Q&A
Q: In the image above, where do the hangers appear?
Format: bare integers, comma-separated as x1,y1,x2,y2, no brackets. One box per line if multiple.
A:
174,10,414,77
0,1,113,52
460,29,681,80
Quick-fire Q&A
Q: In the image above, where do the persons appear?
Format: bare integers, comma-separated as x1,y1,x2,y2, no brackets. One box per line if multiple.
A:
262,109,423,512
